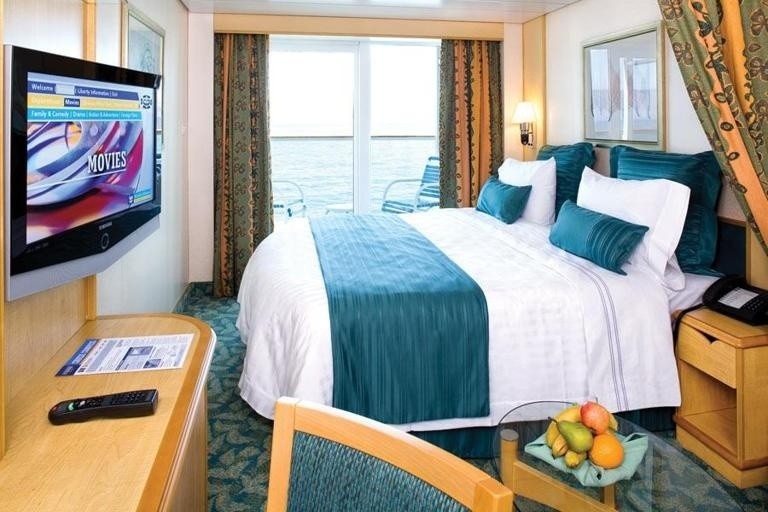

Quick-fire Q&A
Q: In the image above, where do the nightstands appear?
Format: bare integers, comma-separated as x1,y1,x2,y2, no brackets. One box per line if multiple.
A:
671,302,768,491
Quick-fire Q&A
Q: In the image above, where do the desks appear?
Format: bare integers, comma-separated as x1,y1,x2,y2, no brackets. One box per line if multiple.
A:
324,204,354,214
489,397,743,512
0,311,220,511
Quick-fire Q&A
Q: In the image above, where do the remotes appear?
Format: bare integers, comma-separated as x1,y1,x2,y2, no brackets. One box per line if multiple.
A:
48,389,159,426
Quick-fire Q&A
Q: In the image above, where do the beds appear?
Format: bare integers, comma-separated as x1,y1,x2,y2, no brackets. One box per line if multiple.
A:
235,141,749,462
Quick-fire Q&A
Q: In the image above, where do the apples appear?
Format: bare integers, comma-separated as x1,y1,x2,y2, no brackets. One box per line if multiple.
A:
580,402,610,433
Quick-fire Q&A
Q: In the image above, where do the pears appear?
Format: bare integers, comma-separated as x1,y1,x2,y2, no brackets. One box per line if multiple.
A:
556,421,594,452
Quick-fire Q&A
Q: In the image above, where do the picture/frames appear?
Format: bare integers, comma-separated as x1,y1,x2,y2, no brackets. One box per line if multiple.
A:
578,18,667,153
118,1,166,149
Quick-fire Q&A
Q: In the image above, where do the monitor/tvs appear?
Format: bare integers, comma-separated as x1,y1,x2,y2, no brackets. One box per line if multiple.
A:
3,44,164,303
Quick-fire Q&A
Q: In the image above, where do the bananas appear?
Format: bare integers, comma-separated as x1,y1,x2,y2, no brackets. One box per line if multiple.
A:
546,401,618,468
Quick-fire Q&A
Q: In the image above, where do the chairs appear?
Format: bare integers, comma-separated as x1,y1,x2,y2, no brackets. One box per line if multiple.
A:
268,178,309,221
264,392,518,511
381,155,442,214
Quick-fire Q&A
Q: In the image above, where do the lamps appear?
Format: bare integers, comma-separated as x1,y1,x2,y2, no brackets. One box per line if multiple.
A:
510,100,540,148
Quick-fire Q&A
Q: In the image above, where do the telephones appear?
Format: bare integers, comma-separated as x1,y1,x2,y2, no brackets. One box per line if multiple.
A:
702,274,768,325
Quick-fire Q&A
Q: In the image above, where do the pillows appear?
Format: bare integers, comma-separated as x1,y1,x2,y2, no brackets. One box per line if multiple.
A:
535,141,599,222
546,198,651,276
575,163,693,278
474,174,534,227
496,153,559,227
608,144,723,279
